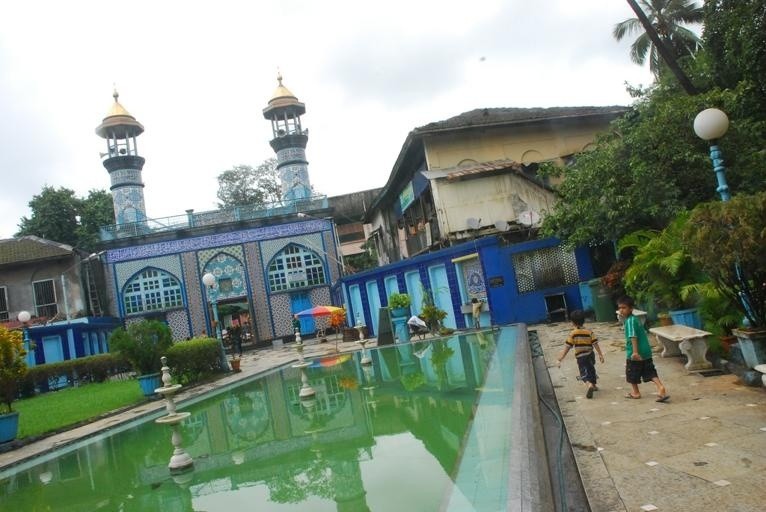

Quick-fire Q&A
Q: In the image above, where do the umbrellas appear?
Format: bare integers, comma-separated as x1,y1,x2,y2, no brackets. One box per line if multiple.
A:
296,305,343,318
305,354,352,368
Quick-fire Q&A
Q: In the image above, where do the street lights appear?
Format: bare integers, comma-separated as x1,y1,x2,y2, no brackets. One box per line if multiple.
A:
693,108,755,327
201,272,229,371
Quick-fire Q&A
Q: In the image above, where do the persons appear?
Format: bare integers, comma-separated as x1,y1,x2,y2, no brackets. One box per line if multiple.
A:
201,329,208,337
616,295,671,402
556,309,604,399
476,332,488,352
229,319,243,357
242,322,250,343
292,314,304,344
471,298,483,328
186,336,191,340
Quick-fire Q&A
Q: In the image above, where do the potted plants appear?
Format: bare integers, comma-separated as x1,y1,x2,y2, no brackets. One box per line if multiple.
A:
386,292,412,317
224,326,246,370
0,324,27,444
599,190,766,371
109,318,174,396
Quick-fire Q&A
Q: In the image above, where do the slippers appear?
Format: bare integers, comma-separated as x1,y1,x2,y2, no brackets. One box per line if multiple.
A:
655,394,670,402
625,393,641,399
587,386,598,397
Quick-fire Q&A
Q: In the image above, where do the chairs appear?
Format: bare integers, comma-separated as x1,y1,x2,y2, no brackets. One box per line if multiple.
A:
410,315,436,340
